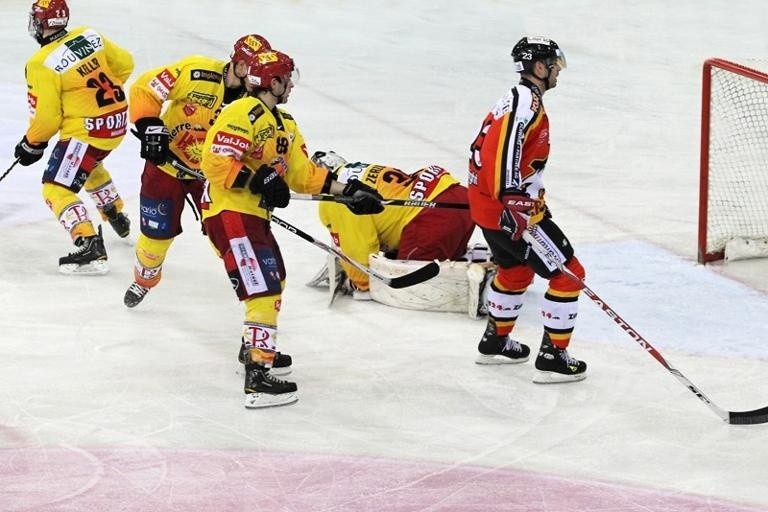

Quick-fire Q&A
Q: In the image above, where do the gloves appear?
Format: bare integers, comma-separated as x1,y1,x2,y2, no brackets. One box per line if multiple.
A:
249,163,291,209
342,180,384,215
139,123,166,167
15,136,48,166
498,189,529,240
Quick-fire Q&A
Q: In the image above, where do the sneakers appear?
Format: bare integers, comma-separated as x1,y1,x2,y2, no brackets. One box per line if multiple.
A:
243,352,296,394
123,281,150,309
106,206,131,238
238,337,291,368
479,331,529,360
533,340,586,375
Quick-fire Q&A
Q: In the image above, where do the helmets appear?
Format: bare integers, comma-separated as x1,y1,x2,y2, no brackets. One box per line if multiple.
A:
310,149,348,175
233,36,271,61
245,48,299,90
27,1,70,37
512,35,567,75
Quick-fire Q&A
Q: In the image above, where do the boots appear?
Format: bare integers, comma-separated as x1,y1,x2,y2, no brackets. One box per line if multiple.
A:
57,224,109,264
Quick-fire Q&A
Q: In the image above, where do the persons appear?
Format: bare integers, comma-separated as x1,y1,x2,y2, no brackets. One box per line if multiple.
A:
199,50,383,397
468,34,588,376
123,35,270,309
310,152,493,318
14,0,135,265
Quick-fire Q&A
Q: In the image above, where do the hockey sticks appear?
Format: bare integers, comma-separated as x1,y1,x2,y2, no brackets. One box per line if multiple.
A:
522,230,768,425
171,159,438,288
